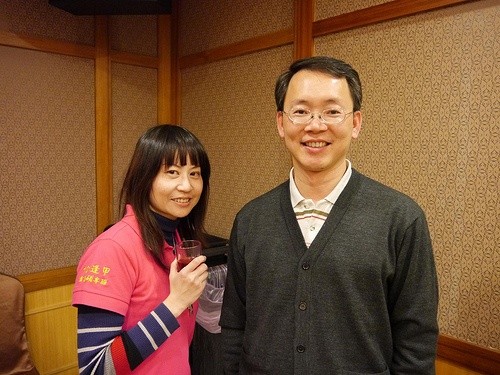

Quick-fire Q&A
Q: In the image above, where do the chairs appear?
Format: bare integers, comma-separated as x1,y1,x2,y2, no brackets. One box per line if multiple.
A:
0,273,41,375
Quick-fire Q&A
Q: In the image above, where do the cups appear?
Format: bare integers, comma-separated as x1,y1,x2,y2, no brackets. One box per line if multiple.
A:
176,239,201,272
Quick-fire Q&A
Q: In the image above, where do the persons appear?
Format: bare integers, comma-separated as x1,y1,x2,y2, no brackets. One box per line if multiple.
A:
212,55,440,375
71,123,211,375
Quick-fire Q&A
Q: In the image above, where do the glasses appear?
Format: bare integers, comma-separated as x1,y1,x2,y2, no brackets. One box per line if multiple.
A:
283,107,353,124
